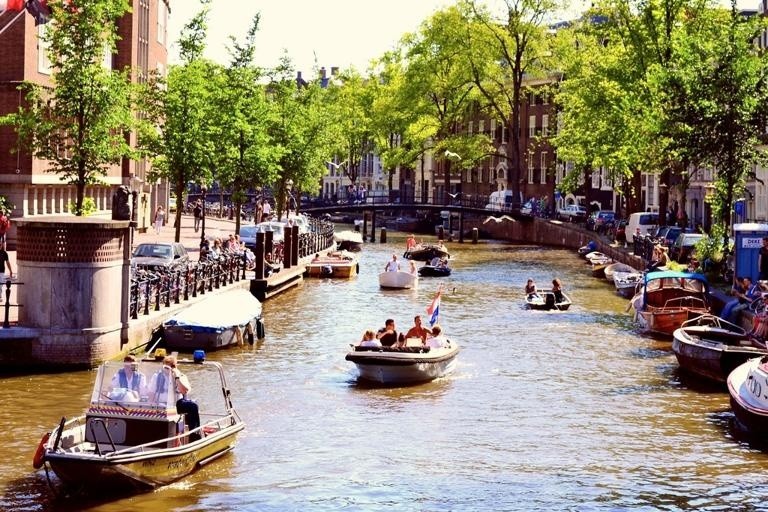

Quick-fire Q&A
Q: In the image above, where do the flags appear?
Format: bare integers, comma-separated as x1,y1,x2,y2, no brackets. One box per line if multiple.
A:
426,283,442,328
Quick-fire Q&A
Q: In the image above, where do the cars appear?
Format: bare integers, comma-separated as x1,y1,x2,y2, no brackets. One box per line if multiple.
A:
238,211,310,256
486,187,709,266
337,198,364,204
301,197,321,202
129,242,190,275
169,198,178,214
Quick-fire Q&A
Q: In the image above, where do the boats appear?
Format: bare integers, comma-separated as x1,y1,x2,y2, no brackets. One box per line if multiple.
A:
346,337,463,382
324,211,467,239
30,335,245,499
308,230,452,290
577,238,767,434
523,288,572,312
157,289,264,348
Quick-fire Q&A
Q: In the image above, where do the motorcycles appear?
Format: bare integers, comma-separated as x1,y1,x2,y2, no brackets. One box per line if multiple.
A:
186,200,252,219
129,250,256,315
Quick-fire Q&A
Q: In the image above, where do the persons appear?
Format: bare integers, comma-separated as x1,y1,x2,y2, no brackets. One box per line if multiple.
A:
147,356,200,431
632,226,768,349
107,352,146,406
384,233,449,274
525,279,539,298
0,243,12,302
361,330,382,347
187,197,271,232
405,316,441,346
345,183,364,205
155,206,165,236
552,279,562,303
376,319,397,351
0,210,11,251
198,234,351,279
